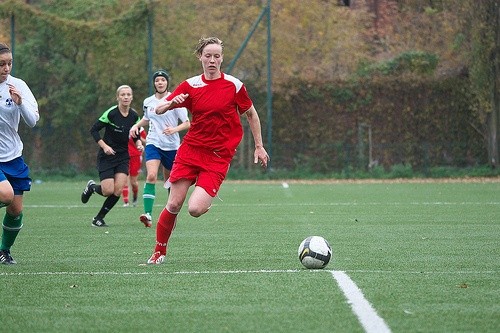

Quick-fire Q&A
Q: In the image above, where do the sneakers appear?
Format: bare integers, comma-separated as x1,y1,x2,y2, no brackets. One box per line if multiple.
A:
122,202,137,208
90,217,111,227
0,250,17,265
80,180,96,204
147,249,166,264
139,212,153,227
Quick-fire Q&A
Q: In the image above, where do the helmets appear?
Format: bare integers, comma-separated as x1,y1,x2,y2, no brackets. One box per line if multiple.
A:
152,70,169,94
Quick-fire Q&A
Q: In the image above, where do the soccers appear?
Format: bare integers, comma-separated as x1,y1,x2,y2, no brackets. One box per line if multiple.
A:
298,235,332,269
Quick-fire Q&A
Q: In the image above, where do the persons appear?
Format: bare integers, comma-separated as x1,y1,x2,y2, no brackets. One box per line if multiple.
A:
0,42,40,264
147,37,270,264
81,71,190,228
